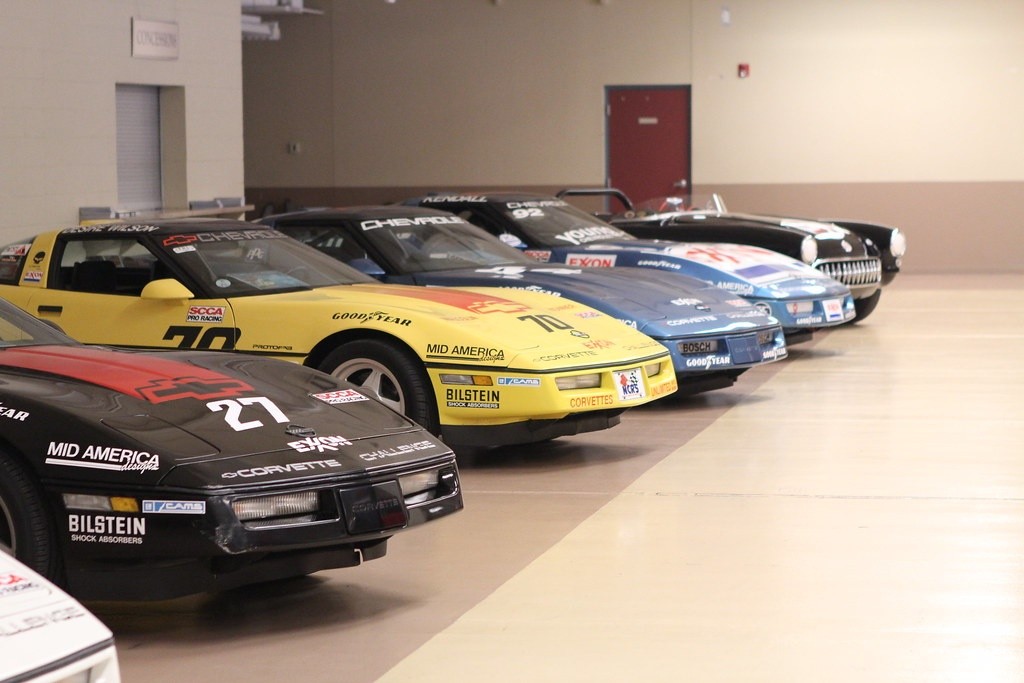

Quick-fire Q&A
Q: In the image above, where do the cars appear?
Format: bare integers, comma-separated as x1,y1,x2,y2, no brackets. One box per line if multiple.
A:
405,192,857,348
1,546,123,683
555,187,910,329
0,294,473,614
250,202,787,403
2,214,676,454
